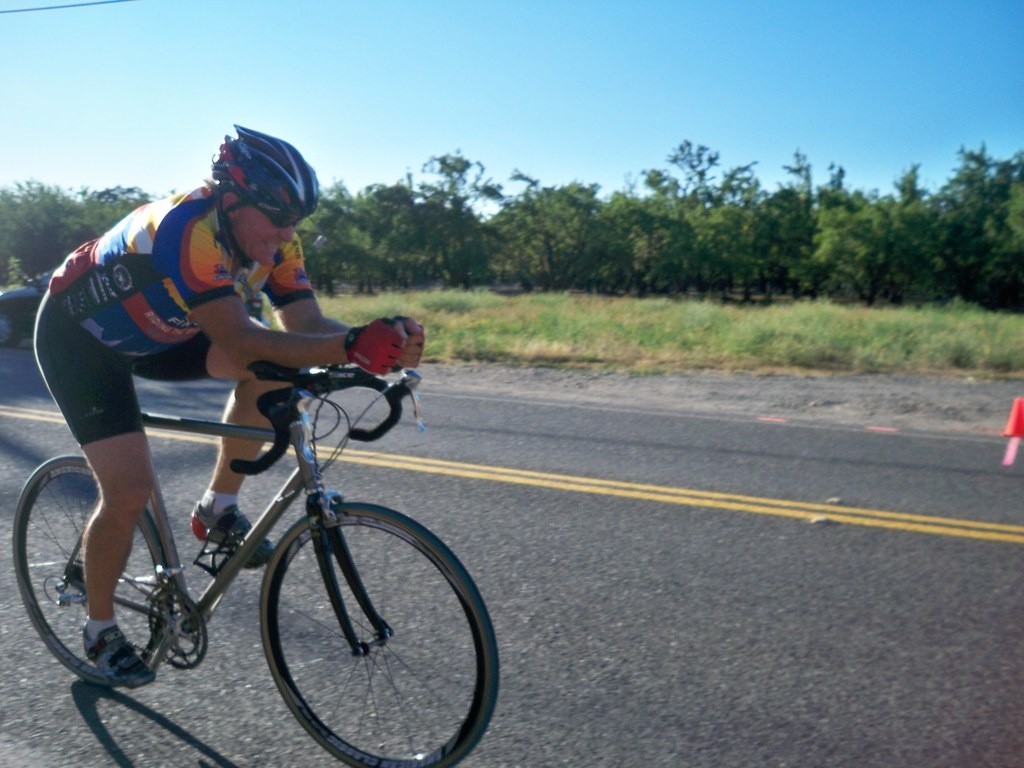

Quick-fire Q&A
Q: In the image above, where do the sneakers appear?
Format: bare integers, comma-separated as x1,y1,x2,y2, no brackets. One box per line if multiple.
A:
82,625,158,686
190,500,276,562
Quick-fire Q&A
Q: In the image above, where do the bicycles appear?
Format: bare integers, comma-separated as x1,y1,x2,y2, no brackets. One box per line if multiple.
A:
13,358,503,768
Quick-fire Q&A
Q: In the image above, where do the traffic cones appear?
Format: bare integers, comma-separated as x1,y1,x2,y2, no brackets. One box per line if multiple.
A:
1002,399,1024,436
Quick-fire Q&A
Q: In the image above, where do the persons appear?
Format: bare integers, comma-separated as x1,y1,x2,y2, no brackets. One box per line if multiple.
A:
35,123,425,689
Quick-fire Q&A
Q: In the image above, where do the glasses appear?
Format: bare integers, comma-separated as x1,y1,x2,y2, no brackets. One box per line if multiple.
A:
250,202,304,227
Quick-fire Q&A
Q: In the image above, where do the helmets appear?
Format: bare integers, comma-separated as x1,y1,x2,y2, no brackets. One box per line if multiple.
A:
212,123,319,218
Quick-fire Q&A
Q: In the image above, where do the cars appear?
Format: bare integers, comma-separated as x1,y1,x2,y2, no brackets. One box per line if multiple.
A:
0,269,52,345
